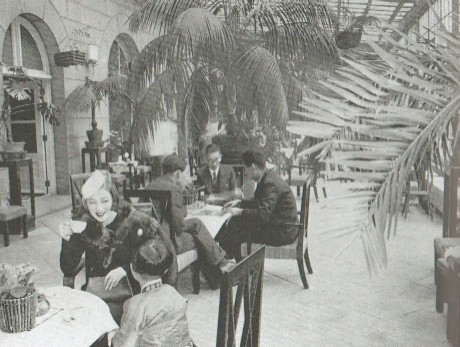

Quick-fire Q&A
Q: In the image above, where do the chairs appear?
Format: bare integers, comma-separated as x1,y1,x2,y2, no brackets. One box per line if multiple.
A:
70,148,327,347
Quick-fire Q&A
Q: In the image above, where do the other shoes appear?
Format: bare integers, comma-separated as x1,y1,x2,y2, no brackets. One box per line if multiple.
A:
220,262,238,275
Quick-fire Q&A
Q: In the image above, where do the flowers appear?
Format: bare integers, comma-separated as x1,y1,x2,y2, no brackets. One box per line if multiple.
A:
0,262,37,300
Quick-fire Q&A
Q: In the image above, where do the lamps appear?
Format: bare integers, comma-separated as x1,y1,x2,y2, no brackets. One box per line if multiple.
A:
85,43,101,68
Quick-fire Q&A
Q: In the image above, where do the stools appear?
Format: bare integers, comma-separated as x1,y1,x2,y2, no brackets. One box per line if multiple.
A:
0,205,29,246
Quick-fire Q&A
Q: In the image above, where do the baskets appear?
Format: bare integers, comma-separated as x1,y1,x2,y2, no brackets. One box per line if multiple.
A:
0,287,50,333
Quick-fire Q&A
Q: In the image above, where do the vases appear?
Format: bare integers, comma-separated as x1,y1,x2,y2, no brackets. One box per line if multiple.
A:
0,288,49,332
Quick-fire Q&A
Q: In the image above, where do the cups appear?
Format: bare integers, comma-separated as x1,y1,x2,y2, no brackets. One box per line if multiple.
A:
70,220,87,233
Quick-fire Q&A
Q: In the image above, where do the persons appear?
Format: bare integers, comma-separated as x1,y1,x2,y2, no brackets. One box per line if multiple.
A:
212,149,301,264
107,240,197,347
194,145,244,200
137,153,237,288
59,169,178,347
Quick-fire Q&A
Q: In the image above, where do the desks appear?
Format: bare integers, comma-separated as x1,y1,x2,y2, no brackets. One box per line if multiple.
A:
0,286,120,347
0,157,35,237
183,201,233,239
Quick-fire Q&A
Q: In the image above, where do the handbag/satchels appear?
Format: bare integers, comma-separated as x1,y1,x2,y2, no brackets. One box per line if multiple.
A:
85,276,134,303
